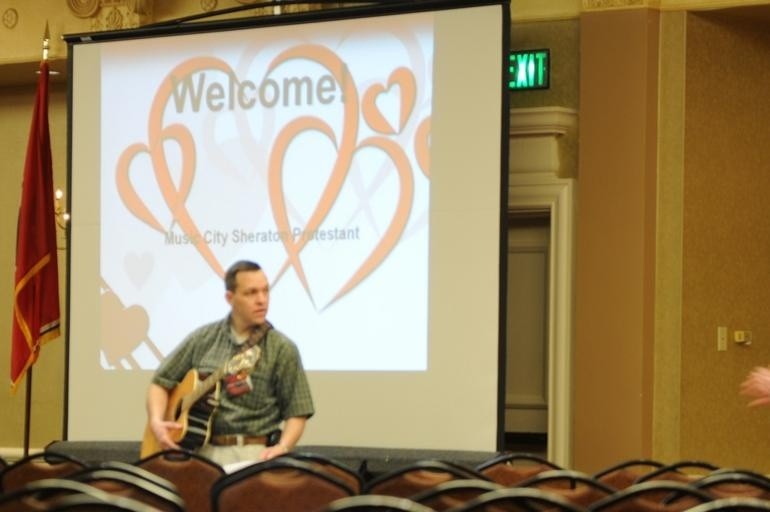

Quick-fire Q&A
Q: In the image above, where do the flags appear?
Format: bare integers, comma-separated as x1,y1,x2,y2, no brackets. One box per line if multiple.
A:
9,60,61,390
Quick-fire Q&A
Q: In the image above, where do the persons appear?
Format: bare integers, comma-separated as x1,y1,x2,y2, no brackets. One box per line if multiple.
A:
738,366,770,407
146,260,316,475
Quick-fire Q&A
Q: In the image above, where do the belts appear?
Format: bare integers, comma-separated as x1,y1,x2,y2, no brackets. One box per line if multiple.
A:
210,435,268,446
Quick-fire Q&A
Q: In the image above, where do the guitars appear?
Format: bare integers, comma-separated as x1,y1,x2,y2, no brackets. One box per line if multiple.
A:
140,342,261,462
